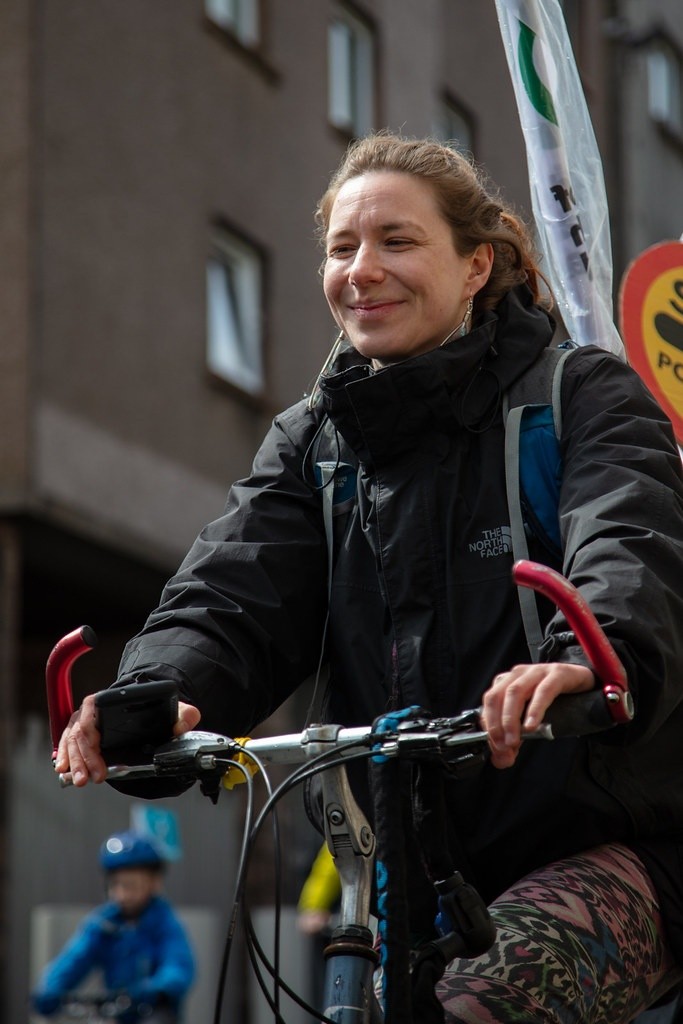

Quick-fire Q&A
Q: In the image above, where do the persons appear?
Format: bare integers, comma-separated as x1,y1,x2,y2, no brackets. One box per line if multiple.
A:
30,833,197,1024
53,136,683,1024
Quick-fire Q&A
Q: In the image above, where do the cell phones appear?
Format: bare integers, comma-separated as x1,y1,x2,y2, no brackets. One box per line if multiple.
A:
94,679,178,769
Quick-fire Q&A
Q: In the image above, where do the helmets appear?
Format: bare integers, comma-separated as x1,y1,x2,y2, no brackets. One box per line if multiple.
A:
95,832,162,873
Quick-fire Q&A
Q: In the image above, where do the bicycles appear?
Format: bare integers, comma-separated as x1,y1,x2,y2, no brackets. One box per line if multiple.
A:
34,985,153,1023
44,562,637,1024
